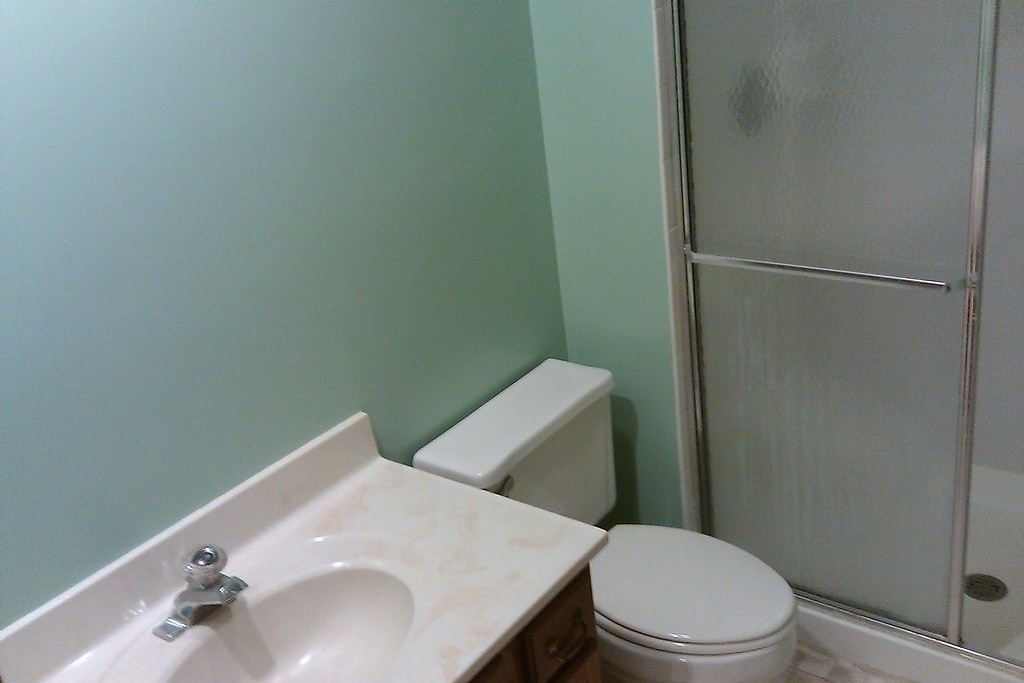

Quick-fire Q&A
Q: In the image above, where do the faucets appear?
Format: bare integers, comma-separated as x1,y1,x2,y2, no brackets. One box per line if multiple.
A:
149,540,249,636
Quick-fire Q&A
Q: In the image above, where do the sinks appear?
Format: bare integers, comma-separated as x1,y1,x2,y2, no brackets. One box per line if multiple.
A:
2,407,610,683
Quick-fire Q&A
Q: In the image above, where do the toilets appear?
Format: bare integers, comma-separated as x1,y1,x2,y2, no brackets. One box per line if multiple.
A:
412,356,801,683
